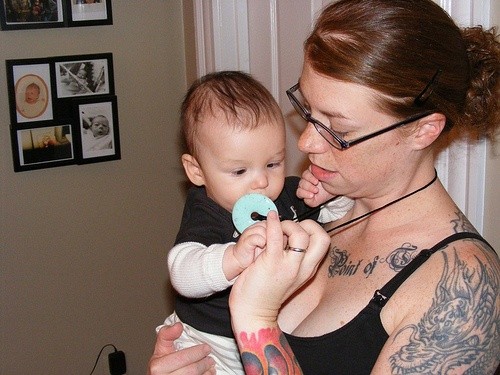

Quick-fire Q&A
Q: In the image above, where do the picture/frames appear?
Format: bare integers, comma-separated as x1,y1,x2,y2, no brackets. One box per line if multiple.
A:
0,0,114,32
4,52,122,173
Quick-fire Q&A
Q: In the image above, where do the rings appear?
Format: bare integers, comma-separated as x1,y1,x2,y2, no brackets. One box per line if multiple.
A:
285,246,307,252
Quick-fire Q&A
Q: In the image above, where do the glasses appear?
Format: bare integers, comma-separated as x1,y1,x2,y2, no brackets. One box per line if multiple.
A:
286,82,440,151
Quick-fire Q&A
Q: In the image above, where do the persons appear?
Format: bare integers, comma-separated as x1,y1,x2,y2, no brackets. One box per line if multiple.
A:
22,83,43,115
83,115,112,152
147,0,500,374
155,70,356,375
61,69,88,95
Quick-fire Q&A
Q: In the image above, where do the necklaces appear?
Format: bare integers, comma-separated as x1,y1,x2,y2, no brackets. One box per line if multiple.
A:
232,166,438,234
